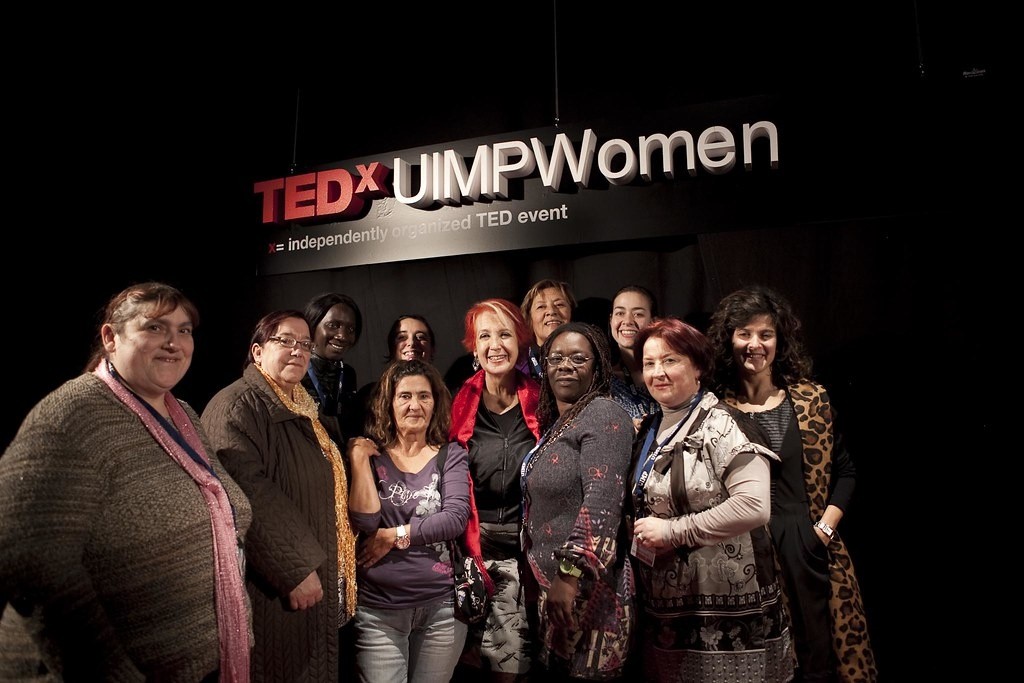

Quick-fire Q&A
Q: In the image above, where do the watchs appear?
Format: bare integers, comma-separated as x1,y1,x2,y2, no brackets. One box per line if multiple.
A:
815,521,835,541
395,524,410,549
559,559,585,580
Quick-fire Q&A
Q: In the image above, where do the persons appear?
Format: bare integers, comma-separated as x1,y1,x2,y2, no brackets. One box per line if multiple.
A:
198,310,357,683
523,278,578,427
603,284,656,433
517,321,636,683
343,313,437,438
707,284,880,683
346,358,472,683
300,290,364,445
620,314,800,683
443,298,544,683
0,282,255,683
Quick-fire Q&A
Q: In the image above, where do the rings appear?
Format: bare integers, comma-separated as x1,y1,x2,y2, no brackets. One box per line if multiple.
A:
638,532,642,541
366,438,369,441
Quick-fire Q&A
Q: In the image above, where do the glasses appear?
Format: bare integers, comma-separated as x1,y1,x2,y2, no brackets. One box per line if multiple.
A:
544,353,595,369
269,335,312,351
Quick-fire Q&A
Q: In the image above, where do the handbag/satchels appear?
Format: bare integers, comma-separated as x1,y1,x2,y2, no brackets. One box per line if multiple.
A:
436,438,493,627
